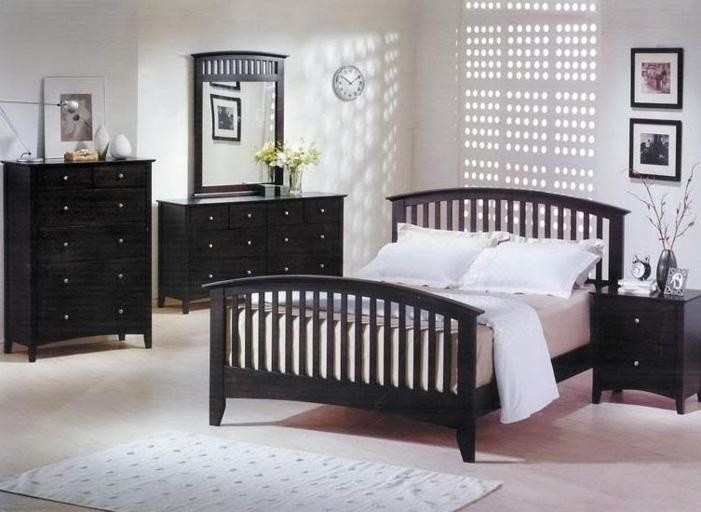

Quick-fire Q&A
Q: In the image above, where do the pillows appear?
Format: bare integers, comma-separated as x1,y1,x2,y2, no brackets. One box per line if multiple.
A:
456,234,604,300
354,221,510,291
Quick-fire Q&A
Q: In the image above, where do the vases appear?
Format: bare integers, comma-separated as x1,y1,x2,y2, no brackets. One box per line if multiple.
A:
656,249,677,291
268,165,275,183
290,168,304,191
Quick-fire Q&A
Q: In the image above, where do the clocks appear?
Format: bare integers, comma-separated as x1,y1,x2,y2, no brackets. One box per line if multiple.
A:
333,65,365,101
631,255,651,281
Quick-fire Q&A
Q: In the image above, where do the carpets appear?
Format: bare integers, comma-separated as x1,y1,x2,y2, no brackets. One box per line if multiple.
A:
0,430,503,512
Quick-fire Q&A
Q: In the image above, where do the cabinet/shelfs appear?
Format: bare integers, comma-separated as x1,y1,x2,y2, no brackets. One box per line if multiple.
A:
589,285,701,414
155,191,348,314
0,158,156,363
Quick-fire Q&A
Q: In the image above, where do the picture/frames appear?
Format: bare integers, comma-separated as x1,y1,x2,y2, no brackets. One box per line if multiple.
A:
664,267,689,296
209,81,240,90
629,118,682,182
630,47,683,109
209,93,241,142
42,75,107,159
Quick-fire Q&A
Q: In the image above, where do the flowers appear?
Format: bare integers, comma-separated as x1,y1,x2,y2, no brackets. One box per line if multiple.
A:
621,162,699,249
255,141,276,168
269,137,319,171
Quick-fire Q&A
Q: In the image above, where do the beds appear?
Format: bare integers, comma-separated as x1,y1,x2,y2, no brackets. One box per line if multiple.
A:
200,187,631,463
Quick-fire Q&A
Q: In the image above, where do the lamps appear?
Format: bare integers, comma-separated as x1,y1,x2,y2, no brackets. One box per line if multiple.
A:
0,98,78,162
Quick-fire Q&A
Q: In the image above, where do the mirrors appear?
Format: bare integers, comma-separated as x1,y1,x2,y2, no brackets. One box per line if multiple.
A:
190,50,289,199
